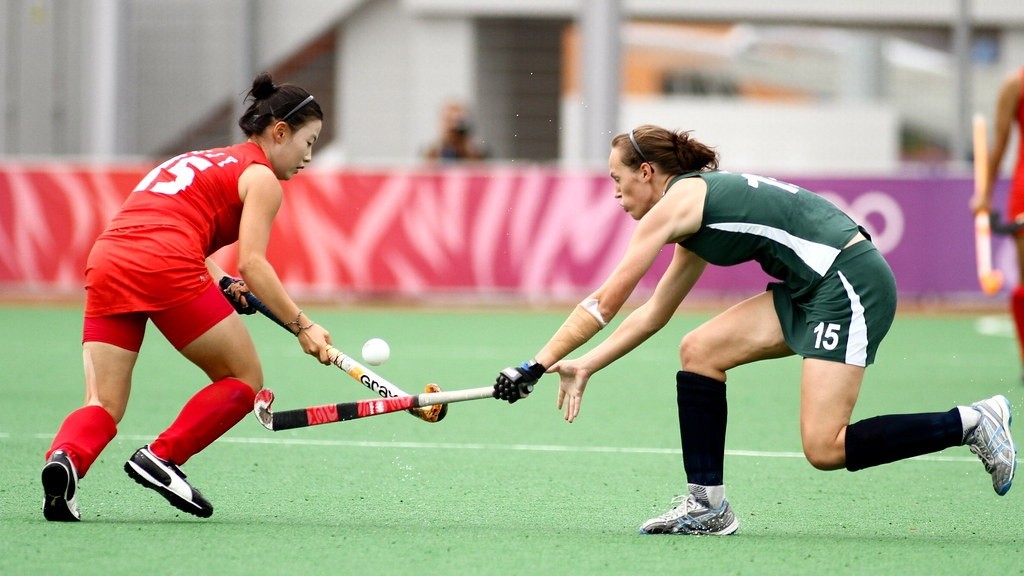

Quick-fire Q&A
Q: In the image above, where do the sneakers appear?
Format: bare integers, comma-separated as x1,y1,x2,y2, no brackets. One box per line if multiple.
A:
638,483,740,537
962,393,1017,496
41,449,81,522
124,444,213,519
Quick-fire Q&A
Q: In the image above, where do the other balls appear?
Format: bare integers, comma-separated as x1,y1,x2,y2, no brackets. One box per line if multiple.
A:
361,337,393,367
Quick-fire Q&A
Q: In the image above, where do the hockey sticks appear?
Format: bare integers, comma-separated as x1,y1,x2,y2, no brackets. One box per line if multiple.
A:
217,275,449,425
253,383,535,434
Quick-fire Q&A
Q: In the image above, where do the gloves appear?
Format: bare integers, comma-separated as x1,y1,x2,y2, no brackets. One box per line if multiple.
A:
493,360,546,404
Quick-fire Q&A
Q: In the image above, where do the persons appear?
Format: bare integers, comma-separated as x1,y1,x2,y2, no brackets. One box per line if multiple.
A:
967,66,1024,368
422,98,493,161
40,72,332,522
491,126,1015,537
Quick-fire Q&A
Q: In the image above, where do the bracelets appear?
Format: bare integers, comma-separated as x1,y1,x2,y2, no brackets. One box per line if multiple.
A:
284,311,315,336
